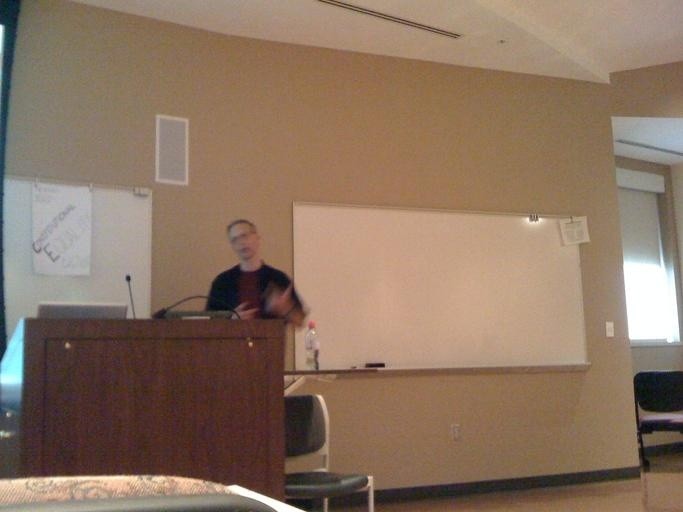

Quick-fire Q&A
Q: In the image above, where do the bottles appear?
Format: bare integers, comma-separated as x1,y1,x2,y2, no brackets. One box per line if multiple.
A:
304,320,321,372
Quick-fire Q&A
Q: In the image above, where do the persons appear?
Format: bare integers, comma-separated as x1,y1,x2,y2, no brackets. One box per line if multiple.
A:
204,218,305,328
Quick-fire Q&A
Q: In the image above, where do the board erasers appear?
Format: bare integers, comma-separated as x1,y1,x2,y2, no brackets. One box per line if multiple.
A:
365,363,385,368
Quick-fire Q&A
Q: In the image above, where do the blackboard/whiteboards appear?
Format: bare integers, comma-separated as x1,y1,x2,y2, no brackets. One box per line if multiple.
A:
292,200,592,377
1,176,153,352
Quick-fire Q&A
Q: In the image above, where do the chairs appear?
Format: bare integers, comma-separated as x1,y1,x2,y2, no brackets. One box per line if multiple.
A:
283,393,376,512
633,370,683,466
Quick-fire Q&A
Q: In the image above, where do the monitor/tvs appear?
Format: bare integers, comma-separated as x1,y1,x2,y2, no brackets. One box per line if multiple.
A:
36,302,128,319
163,310,232,320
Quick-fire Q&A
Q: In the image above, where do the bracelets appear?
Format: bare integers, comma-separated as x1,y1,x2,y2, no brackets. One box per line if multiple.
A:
281,309,295,321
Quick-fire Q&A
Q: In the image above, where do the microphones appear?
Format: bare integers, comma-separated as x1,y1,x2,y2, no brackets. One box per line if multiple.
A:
150,295,242,320
125,275,135,318
